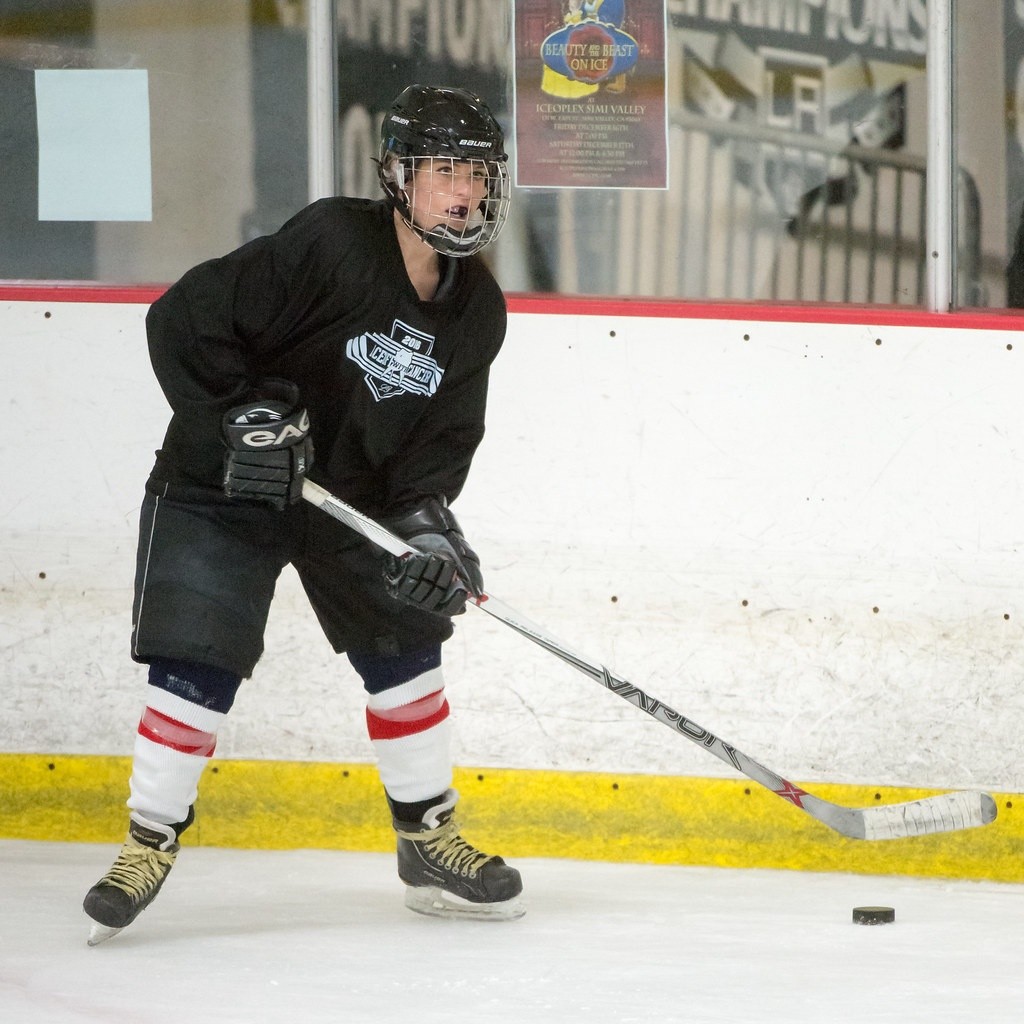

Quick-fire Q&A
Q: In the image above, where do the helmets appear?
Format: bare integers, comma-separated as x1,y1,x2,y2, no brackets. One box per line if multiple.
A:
377,85,513,258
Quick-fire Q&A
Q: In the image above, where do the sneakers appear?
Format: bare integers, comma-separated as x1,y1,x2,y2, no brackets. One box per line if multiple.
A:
83,804,195,947
384,786,527,922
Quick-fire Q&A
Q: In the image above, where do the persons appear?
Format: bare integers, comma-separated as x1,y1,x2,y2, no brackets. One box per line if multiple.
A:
85,84,527,946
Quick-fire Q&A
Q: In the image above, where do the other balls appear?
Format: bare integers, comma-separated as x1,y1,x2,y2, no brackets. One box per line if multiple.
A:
851,906,897,927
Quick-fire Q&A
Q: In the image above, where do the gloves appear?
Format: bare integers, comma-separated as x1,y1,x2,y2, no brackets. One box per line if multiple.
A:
367,497,484,618
219,400,315,512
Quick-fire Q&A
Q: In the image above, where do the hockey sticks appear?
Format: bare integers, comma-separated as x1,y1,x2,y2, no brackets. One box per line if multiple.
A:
298,475,999,843
